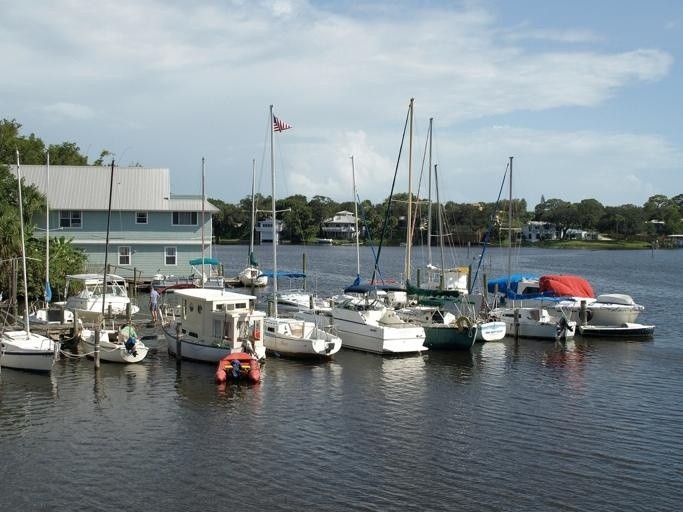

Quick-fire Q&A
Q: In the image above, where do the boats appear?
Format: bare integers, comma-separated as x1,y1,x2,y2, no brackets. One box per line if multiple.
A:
63,272,141,321
79,326,151,365
213,352,262,384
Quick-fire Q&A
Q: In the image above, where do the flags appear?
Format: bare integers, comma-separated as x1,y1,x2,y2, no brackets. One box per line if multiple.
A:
271,114,292,133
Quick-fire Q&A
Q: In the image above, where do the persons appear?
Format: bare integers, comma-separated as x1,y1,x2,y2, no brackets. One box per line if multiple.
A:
146,286,160,322
120,323,138,356
30,286,39,311
37,288,48,309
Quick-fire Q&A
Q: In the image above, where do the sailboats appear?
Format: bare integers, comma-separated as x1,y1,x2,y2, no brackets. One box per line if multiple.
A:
238,99,655,358
2,149,61,372
23,151,82,347
155,156,266,364
